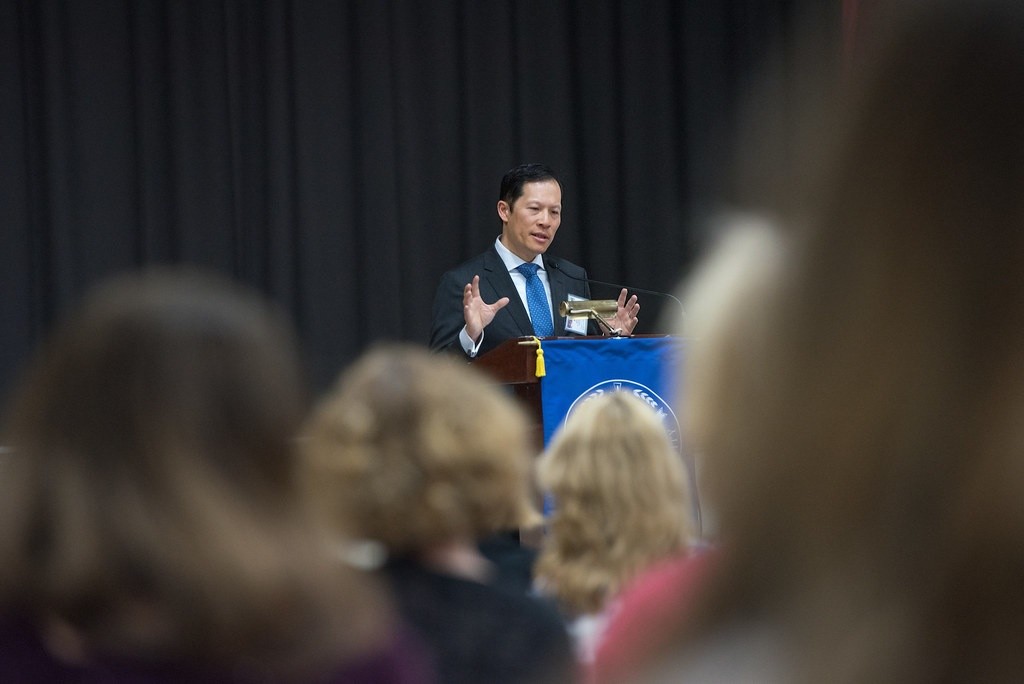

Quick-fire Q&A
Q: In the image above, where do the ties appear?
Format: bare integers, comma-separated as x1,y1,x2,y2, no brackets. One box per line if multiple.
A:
515,263,554,337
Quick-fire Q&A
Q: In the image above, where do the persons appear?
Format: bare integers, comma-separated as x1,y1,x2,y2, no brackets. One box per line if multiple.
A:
306,338,584,684
588,3,1024,684
0,259,448,684
429,164,643,360
538,384,709,660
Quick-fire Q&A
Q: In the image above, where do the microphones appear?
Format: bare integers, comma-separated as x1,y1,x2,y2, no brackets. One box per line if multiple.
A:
546,257,684,318
